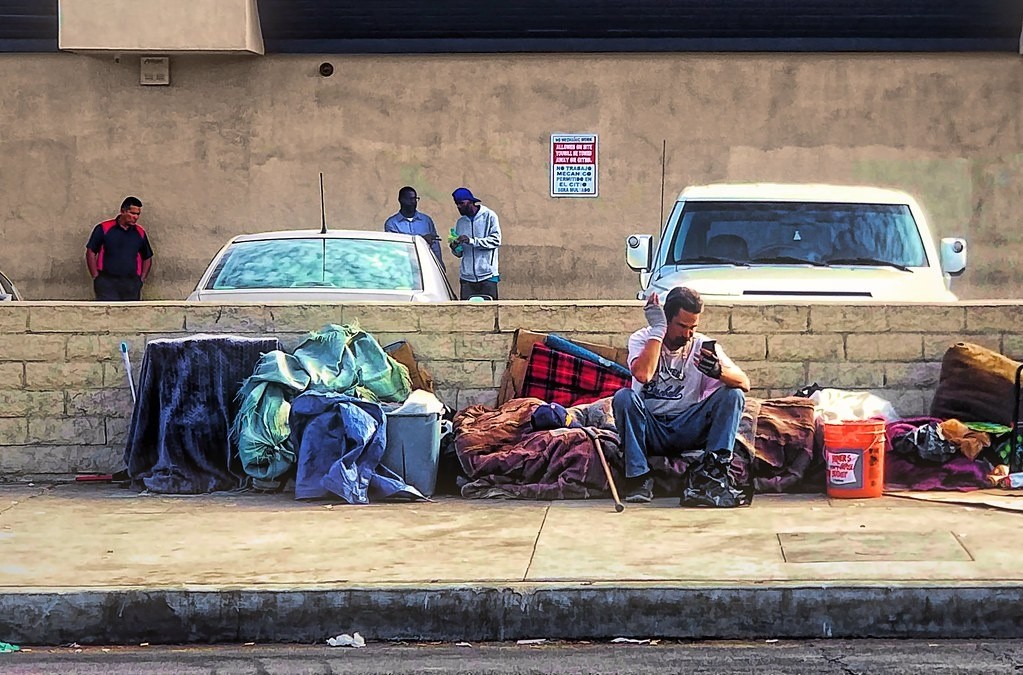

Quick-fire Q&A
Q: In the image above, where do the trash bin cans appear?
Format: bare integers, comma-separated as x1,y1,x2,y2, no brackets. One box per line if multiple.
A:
373,402,447,498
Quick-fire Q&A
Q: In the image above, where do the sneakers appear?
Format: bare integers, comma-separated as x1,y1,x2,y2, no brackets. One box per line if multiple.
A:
689,463,743,505
624,473,651,501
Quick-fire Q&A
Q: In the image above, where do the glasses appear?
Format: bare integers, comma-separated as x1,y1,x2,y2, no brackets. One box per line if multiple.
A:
401,195,422,202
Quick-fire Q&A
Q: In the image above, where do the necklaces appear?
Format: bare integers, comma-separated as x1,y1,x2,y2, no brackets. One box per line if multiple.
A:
661,343,687,379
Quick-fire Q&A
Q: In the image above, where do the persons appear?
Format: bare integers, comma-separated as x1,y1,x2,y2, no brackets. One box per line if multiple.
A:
85,196,154,300
611,285,750,509
384,186,447,275
449,187,500,300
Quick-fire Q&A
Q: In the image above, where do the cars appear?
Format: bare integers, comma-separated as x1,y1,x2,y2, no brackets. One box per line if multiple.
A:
621,182,970,303
184,171,491,301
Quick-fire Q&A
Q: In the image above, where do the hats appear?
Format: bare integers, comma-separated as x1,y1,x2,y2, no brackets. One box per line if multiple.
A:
451,187,480,203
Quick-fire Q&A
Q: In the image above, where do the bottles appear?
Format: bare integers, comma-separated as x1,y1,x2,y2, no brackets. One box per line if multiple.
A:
449,228,464,253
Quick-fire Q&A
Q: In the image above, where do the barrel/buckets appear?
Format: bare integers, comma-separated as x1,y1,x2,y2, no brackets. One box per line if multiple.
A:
380,404,443,498
821,418,884,498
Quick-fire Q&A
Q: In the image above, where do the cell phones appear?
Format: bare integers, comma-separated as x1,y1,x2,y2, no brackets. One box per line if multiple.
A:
700,340,716,356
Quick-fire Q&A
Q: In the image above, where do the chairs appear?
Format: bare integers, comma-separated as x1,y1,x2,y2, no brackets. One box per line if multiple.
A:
702,233,749,265
832,228,880,262
